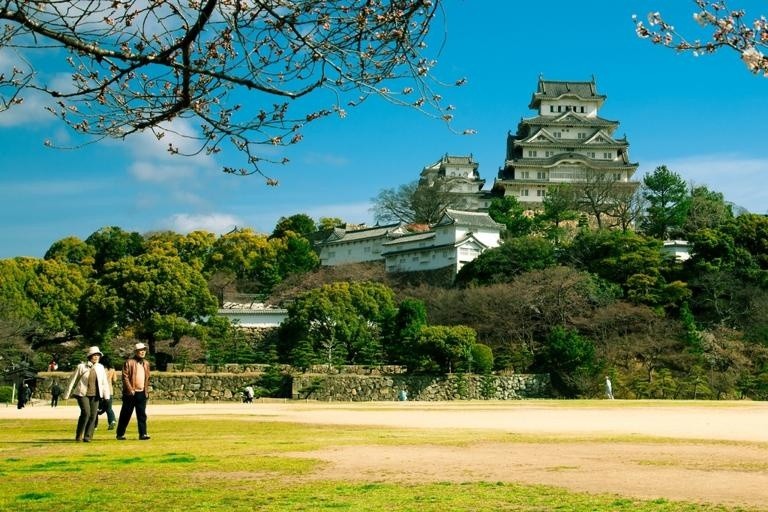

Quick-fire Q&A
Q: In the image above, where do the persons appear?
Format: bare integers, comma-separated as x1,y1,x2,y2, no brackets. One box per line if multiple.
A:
94,355,117,431
599,375,615,401
16,358,60,409
115,341,151,440
61,346,111,443
398,388,409,401
242,383,254,404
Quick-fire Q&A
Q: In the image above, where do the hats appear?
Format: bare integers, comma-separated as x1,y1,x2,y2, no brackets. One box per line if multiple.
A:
134,342,148,350
88,346,104,358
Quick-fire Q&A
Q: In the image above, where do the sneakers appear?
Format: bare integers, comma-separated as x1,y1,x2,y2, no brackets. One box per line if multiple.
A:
76,433,82,441
140,435,150,440
84,436,91,441
116,435,125,439
108,421,116,430
